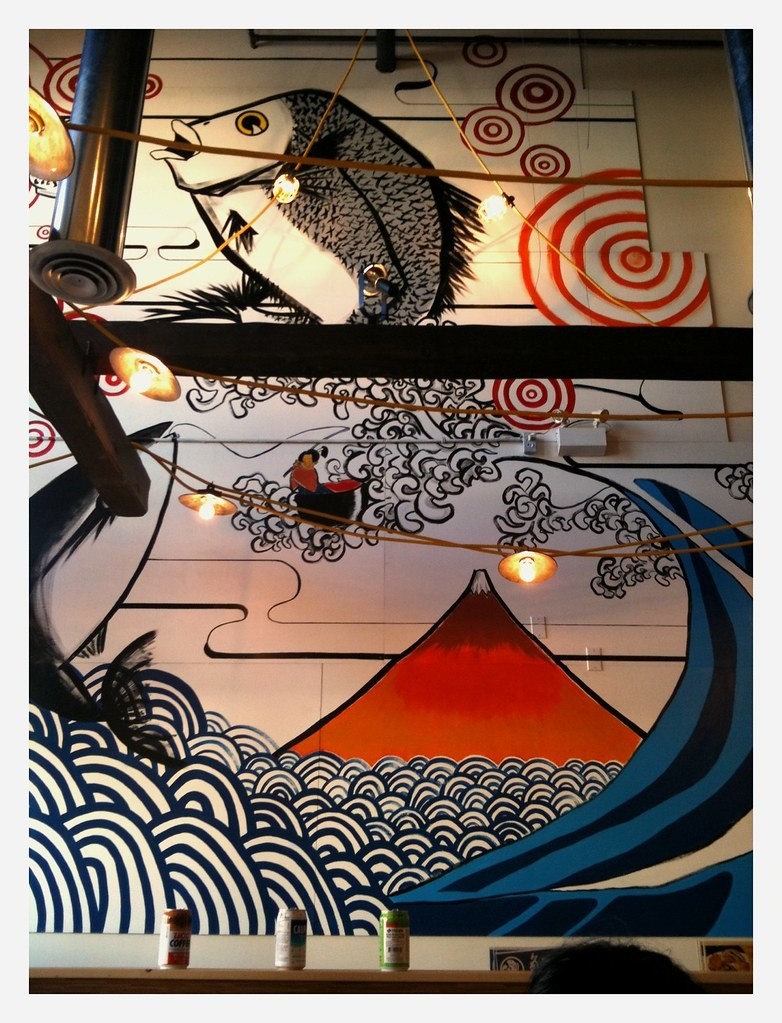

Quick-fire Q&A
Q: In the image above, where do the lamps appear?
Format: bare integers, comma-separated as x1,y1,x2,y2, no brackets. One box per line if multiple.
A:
29,85,76,181
355,260,400,326
176,481,239,521
109,345,183,403
496,547,558,585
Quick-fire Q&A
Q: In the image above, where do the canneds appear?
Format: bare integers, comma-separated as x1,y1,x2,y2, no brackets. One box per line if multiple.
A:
158,907,191,971
378,912,410,973
274,908,308,971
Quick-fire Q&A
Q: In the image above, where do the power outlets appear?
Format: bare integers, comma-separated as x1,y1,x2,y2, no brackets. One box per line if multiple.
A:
521,430,539,455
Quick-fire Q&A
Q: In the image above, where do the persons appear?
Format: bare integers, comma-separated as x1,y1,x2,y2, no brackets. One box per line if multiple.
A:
527,940,702,994
